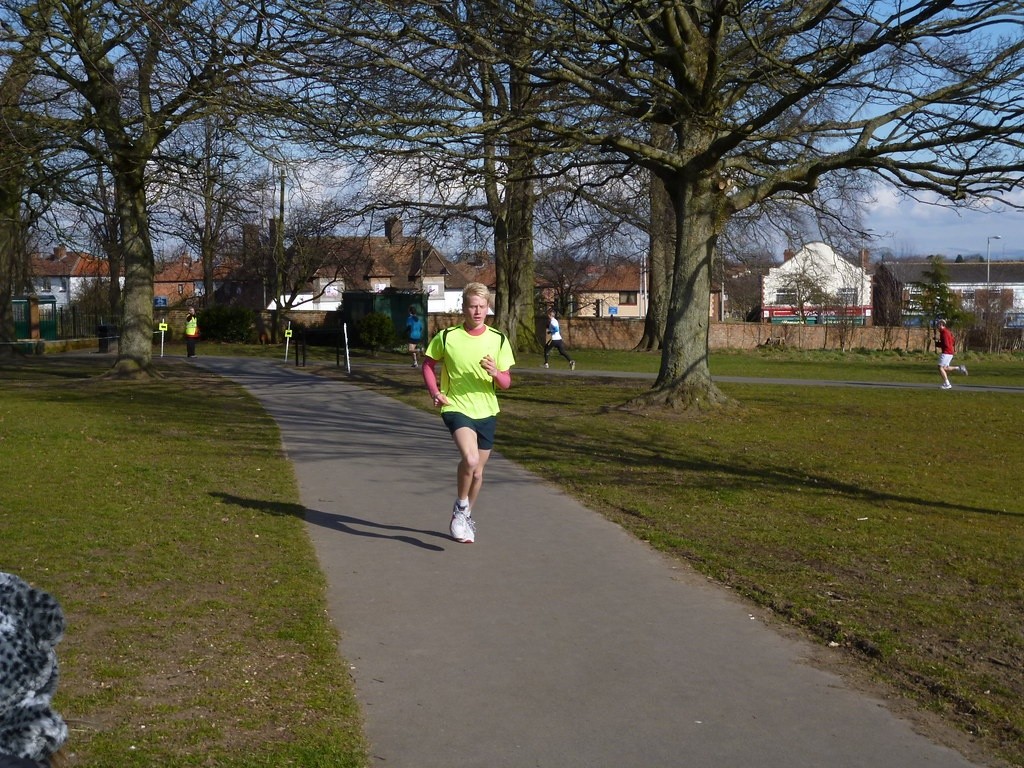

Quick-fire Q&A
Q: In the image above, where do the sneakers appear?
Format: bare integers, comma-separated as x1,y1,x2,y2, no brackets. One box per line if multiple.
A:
450,513,475,544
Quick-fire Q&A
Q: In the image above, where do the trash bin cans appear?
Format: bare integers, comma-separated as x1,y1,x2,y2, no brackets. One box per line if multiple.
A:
97,325,119,352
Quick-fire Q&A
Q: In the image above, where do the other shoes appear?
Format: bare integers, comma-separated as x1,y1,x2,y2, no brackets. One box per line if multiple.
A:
540,363,549,369
411,363,418,367
419,348,424,357
188,355,197,358
568,361,576,371
959,365,969,376
941,384,952,389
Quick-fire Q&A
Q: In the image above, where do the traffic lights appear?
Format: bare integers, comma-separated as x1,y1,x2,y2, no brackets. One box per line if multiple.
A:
593,299,603,317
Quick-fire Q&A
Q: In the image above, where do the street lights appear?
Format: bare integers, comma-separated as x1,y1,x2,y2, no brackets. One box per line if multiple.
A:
862,228,875,326
987,236,1001,302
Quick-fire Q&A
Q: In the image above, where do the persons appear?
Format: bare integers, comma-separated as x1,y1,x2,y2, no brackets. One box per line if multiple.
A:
543,309,575,370
422,283,516,544
185,307,200,358
404,307,424,368
934,320,968,389
